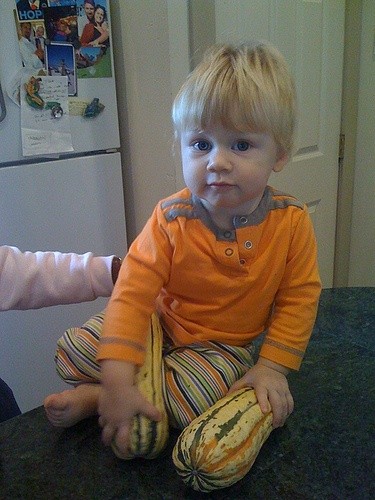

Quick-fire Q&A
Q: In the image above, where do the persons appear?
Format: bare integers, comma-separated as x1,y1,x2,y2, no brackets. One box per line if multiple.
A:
0,246,125,424
16,0,111,71
43,41,322,461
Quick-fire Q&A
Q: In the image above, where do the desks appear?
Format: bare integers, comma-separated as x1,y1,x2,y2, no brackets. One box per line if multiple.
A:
0,286,375,500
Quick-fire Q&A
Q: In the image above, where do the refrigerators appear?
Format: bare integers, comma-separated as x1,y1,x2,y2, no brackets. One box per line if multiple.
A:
0,0,130,413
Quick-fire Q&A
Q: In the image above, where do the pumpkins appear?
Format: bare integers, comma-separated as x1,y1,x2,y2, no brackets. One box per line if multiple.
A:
171,386,273,493
111,310,169,459
